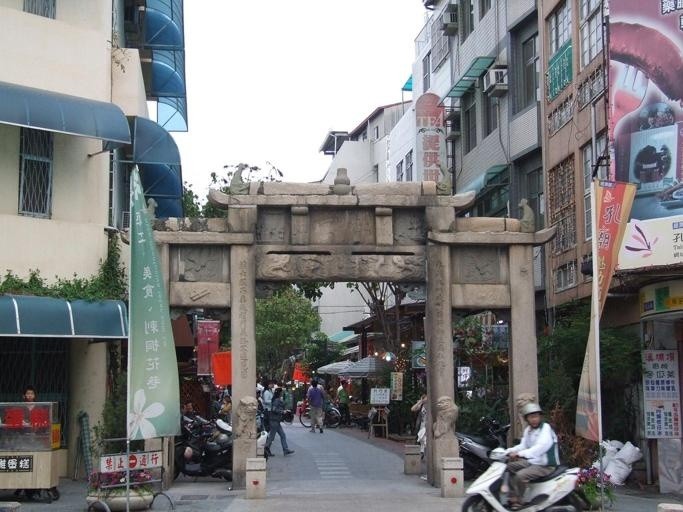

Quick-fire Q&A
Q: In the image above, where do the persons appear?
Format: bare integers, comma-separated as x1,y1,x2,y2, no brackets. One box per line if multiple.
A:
409,389,428,481
17,386,47,500
502,401,562,512
179,376,357,460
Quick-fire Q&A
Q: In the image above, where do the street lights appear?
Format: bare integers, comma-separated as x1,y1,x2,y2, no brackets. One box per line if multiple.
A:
310,337,329,392
341,326,368,405
291,347,306,393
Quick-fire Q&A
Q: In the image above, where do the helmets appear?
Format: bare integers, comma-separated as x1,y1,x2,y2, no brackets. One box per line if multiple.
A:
209,401,220,410
523,403,542,416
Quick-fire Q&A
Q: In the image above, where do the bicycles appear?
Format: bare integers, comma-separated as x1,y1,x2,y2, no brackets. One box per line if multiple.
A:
299,402,342,428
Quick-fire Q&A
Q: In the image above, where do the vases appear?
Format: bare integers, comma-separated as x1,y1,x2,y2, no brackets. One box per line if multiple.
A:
582,492,608,506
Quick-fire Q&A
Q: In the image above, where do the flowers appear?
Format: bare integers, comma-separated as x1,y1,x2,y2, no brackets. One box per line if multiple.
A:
88,464,153,506
574,467,617,506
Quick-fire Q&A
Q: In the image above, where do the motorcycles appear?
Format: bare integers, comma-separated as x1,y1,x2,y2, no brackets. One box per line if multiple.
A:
455,415,511,479
172,398,293,483
462,446,588,511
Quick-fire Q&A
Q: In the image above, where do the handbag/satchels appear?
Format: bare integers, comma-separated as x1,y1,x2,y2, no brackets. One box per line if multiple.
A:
416,413,422,431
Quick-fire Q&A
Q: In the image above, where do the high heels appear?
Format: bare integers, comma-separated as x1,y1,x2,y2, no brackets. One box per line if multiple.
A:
283,449,294,456
264,448,274,456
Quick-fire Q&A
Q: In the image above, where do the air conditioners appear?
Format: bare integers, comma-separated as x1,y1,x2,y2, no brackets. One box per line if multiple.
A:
484,68,506,89
438,13,456,30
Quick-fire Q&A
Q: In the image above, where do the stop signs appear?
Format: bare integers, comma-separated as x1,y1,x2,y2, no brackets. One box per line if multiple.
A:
127,454,136,468
